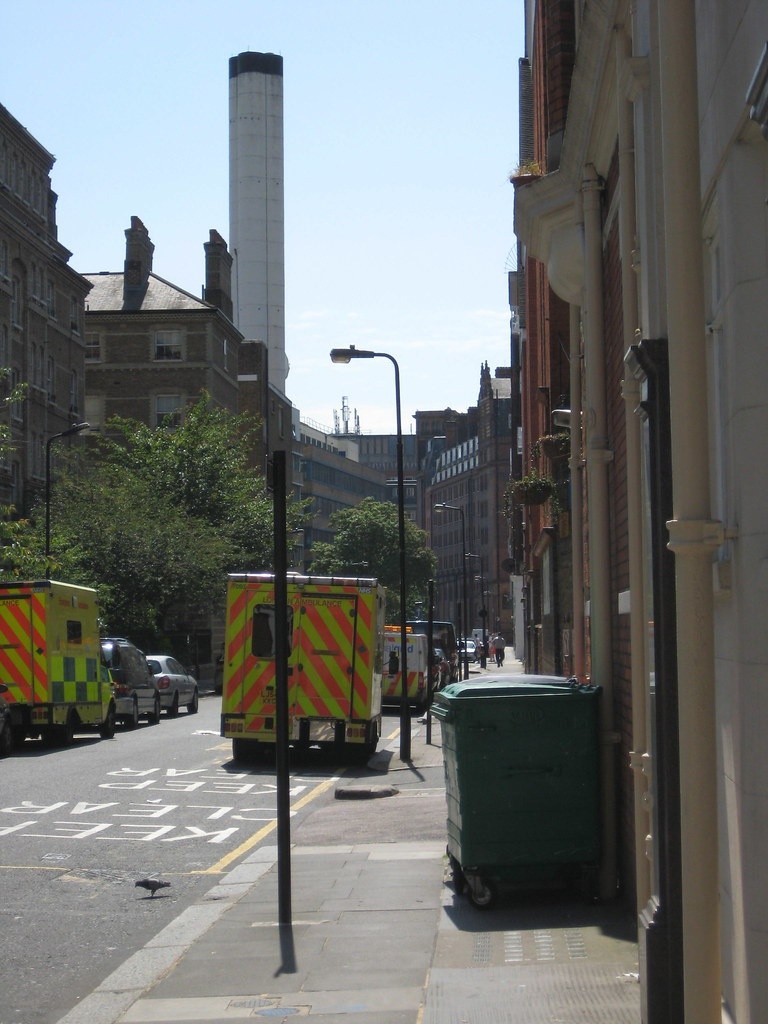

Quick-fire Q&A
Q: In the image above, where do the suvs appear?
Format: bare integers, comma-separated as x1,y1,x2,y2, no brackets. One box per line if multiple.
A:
100,638,160,729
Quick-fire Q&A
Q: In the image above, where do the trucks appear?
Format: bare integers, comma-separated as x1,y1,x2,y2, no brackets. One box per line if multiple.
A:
0,580,116,747
220,572,386,763
381,626,439,715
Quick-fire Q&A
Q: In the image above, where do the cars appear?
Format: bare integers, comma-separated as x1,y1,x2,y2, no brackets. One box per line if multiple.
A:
456,637,485,663
146,655,198,717
435,648,450,691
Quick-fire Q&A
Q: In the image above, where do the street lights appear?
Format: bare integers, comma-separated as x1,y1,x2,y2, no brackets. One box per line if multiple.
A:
434,503,469,680
244,529,304,573
44,423,90,581
474,576,497,633
330,345,411,759
464,553,488,657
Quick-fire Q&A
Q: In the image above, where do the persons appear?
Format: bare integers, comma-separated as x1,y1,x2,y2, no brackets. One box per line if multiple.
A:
492,632,505,668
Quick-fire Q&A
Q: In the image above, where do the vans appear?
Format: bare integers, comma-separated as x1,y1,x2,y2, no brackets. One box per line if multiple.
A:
406,621,460,681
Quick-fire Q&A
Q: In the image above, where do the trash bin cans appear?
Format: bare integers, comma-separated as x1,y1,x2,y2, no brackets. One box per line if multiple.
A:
429,674,606,908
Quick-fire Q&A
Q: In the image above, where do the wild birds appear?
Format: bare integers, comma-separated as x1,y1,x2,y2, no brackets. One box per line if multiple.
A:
135,879,170,898
